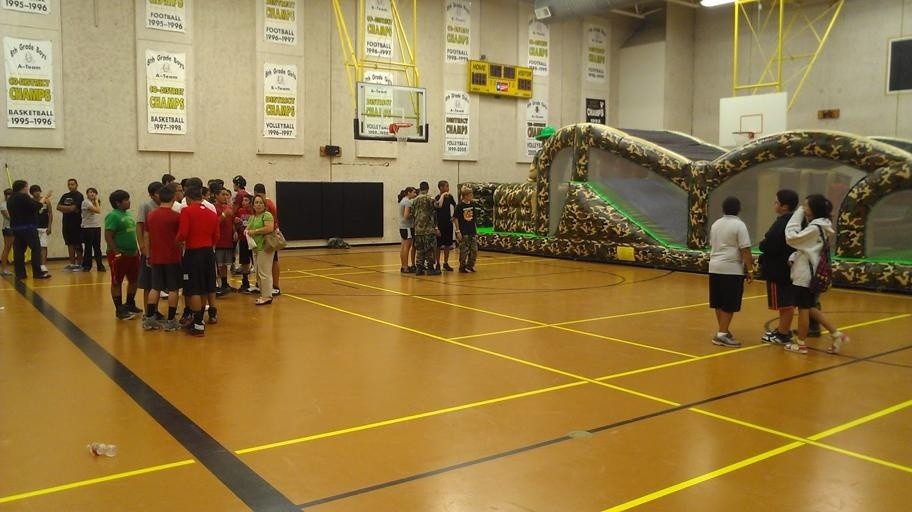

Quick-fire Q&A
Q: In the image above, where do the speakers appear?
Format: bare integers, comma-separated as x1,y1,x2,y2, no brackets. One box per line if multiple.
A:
534,5,551,20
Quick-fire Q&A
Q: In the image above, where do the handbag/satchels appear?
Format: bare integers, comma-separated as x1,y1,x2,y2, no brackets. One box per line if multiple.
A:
264,228,287,251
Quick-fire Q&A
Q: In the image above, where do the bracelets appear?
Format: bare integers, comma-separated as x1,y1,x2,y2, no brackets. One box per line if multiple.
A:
747,270,754,273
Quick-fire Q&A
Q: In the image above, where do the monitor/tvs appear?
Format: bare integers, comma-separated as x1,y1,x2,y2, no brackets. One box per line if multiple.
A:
887,36,912,94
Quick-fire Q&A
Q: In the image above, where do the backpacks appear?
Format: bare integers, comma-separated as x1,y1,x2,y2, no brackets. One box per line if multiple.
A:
808,224,833,294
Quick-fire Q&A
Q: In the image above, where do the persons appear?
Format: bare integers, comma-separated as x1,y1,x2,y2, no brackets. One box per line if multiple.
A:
396,181,480,276
57,178,106,272
758,189,810,346
2,180,53,279
706,196,755,347
105,173,281,336
783,193,851,355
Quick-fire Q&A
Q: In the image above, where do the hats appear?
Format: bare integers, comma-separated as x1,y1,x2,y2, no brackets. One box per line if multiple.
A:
233,175,246,186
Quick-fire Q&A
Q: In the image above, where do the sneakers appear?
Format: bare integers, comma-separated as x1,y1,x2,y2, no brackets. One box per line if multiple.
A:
0,264,106,279
216,266,280,304
116,301,217,337
712,326,849,354
401,263,475,275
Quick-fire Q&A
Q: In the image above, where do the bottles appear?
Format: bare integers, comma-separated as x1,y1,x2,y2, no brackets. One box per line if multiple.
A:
84,442,119,460
